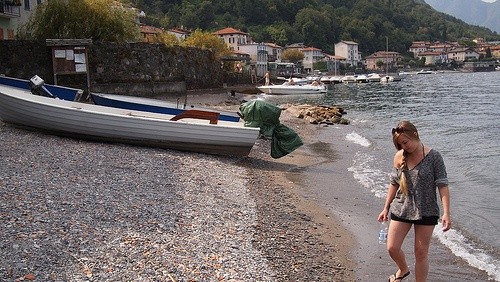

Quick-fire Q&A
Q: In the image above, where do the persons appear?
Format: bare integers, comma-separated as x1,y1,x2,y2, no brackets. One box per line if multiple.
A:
378,121,451,282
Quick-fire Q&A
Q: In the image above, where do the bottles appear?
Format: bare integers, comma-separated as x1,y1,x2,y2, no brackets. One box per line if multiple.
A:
379,220,388,245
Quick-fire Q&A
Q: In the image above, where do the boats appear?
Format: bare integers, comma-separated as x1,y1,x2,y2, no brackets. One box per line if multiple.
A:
495,65,500,71
277,72,382,83
398,72,413,75
416,69,432,74
257,74,329,95
0,72,241,122
0,84,304,158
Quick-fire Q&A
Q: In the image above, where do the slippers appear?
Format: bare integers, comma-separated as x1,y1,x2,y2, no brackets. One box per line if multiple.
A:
388,270,410,282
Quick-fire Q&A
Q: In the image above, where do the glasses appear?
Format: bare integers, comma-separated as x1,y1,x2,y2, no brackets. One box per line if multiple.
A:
392,127,411,134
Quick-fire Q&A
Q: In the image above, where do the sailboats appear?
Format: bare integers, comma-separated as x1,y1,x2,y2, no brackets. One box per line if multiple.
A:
381,36,401,81
